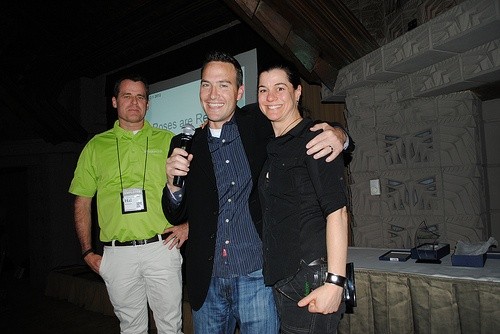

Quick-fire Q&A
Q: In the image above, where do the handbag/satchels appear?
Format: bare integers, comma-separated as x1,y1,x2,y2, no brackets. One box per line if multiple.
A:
327,262,358,307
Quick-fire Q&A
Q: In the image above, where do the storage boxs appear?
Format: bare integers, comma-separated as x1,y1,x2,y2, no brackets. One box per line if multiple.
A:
379,242,488,268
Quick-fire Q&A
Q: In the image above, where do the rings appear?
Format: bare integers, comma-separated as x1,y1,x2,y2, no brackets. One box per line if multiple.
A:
175,237,180,241
329,144,334,152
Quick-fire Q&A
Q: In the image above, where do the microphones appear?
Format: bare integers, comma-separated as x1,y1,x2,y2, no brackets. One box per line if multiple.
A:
174,124,195,188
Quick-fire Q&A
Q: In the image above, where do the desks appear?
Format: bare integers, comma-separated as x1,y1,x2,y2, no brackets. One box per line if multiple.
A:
338,247,500,334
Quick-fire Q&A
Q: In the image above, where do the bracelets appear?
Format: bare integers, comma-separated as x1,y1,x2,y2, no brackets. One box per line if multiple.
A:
82,250,92,258
324,273,346,288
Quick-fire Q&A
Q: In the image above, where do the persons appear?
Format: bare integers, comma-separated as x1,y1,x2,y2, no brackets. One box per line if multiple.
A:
201,60,350,334
69,72,188,334
162,54,356,334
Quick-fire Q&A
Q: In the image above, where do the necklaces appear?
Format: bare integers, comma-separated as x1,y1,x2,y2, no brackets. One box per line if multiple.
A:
279,115,303,135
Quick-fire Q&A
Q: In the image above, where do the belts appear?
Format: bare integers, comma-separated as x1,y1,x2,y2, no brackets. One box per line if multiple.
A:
103,232,173,246
308,258,326,266
